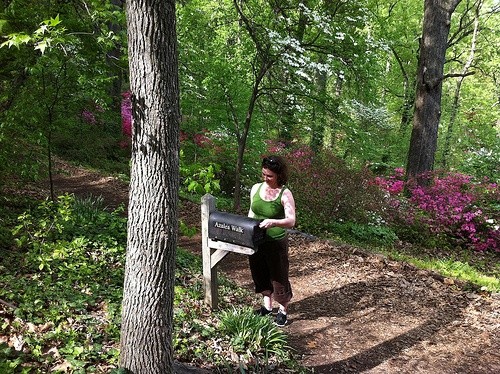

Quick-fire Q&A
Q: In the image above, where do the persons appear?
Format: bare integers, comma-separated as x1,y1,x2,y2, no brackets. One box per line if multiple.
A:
246,156,296,327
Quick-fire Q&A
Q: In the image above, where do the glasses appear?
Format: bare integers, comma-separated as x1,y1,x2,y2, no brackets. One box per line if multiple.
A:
265,157,275,165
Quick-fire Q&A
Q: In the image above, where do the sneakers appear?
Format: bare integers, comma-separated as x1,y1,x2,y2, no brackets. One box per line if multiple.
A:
273,312,288,327
253,306,273,316
